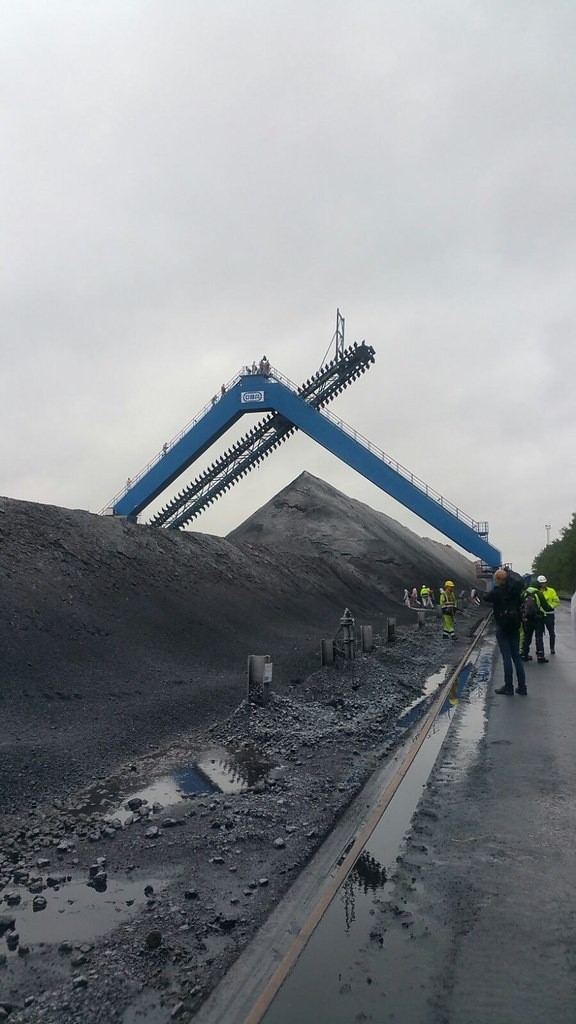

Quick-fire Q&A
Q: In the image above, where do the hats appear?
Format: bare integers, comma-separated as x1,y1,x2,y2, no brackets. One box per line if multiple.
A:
537,575,547,583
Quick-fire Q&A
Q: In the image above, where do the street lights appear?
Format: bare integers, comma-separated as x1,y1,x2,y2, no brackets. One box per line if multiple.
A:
545,524,552,544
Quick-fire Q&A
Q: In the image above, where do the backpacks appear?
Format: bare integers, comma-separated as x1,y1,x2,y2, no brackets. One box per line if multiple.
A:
494,585,521,633
520,590,542,623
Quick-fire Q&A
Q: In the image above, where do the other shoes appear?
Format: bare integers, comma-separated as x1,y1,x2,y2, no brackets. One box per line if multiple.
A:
442,637,449,639
521,654,533,662
515,685,527,695
424,606,427,609
451,637,458,640
432,608,435,609
494,684,515,696
551,649,555,654
537,655,549,664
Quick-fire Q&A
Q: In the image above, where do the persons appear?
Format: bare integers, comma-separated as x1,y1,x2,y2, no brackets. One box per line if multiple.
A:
440,581,458,640
471,566,527,696
403,585,476,609
521,575,560,664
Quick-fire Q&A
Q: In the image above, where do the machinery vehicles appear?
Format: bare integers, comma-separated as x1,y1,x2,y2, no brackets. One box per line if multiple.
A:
146,307,376,532
98,355,514,592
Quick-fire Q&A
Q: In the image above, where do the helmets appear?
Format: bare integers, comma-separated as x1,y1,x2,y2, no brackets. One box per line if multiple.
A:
528,578,539,587
422,585,426,588
445,581,455,588
522,573,532,582
